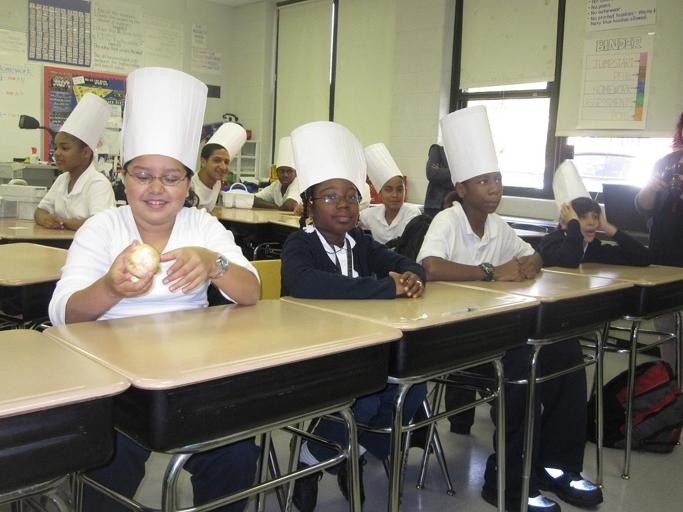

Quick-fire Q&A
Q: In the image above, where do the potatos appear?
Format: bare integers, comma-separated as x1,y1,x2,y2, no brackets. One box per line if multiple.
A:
125,244,160,279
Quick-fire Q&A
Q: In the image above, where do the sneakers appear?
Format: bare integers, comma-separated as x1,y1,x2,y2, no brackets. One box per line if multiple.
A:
338,455,367,501
290,437,323,512
482,455,603,512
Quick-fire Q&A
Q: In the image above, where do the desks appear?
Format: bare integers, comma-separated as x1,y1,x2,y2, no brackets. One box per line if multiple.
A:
0,205,683,512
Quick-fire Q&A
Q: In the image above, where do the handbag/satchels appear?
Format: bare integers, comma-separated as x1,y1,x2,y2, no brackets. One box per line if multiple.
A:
588,360,683,452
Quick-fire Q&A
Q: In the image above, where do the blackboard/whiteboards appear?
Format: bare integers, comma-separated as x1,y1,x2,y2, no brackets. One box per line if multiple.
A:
44,66,128,162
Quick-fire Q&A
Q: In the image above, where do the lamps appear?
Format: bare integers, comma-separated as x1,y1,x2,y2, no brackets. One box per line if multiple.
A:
18,115,55,140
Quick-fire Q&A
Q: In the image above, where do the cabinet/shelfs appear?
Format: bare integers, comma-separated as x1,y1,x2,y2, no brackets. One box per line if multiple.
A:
196,141,259,185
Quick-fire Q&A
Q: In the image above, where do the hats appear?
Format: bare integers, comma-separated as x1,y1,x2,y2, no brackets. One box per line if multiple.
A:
553,161,592,207
121,67,208,175
207,123,247,161
440,106,500,187
58,92,112,150
276,121,403,194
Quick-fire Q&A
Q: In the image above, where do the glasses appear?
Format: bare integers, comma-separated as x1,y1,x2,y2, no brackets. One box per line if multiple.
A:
126,171,186,186
311,194,360,203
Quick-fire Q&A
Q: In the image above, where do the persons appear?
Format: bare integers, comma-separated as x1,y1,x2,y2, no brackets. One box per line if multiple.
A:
36,92,116,316
281,120,427,511
186,122,311,260
635,112,683,386
538,159,649,267
417,104,604,511
48,66,262,510
358,143,464,263
